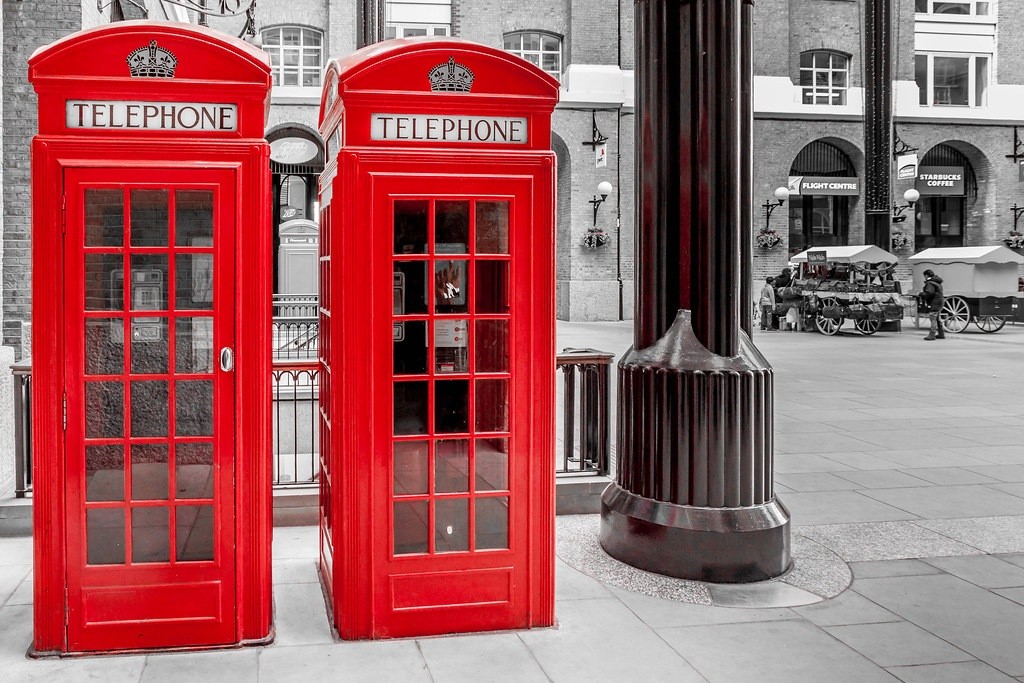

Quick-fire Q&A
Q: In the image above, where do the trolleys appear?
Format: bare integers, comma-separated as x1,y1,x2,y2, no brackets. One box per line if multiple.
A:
791,245,903,336
902,246,1024,334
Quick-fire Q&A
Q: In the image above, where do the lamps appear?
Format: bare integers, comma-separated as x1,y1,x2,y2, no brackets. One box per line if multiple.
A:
892,189,920,223
589,181,612,226
762,187,789,227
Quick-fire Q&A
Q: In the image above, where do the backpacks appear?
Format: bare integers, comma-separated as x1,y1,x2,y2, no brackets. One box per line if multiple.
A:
848,297,867,319
882,275,896,293
865,296,883,320
882,297,904,320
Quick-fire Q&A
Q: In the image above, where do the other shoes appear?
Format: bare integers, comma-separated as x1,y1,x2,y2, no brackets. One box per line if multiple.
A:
761,327,766,330
767,327,777,331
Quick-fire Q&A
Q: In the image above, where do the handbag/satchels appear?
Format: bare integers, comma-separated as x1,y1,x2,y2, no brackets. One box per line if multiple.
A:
804,318,816,326
834,304,849,318
822,306,834,318
916,296,932,313
786,307,798,323
777,287,793,298
776,299,795,315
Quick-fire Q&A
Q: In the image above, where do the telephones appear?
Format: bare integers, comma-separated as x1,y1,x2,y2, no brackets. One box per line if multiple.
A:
110,269,163,344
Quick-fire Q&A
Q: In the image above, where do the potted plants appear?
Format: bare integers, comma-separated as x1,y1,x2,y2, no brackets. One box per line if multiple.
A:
892,232,914,251
579,228,612,252
1001,232,1024,250
756,227,785,251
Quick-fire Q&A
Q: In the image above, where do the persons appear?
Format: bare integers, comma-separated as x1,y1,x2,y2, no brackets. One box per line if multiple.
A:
917,269,946,340
771,268,792,329
761,276,777,331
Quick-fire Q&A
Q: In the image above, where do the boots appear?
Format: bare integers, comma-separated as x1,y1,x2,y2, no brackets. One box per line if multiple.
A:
924,330,936,340
935,329,945,339
